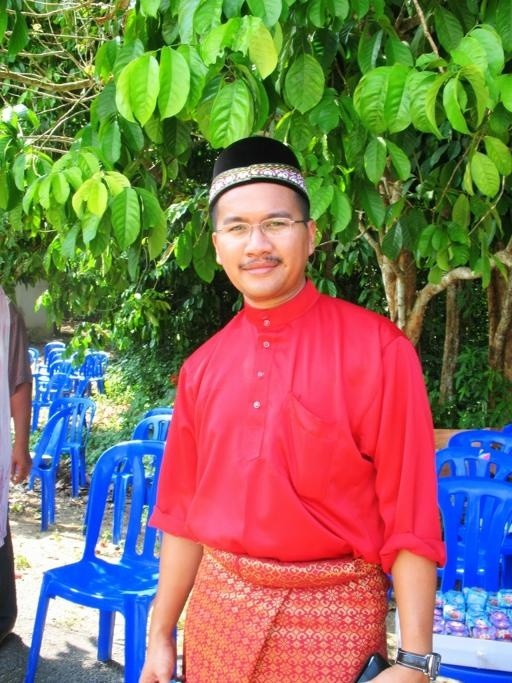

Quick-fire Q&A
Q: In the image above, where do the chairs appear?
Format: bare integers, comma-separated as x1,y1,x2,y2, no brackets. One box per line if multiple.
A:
377,427,512,682
23,342,113,530
104,408,176,546
18,440,180,683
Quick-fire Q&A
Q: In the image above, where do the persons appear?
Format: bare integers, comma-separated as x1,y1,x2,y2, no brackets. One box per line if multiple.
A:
0,285,33,651
137,138,447,683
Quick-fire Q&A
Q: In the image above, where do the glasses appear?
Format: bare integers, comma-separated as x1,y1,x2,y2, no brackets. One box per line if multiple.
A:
213,217,314,242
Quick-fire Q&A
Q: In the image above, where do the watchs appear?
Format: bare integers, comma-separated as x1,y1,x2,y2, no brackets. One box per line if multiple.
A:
395,648,441,681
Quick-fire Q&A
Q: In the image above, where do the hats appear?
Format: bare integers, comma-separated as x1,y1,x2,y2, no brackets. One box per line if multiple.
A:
208,135,311,214
